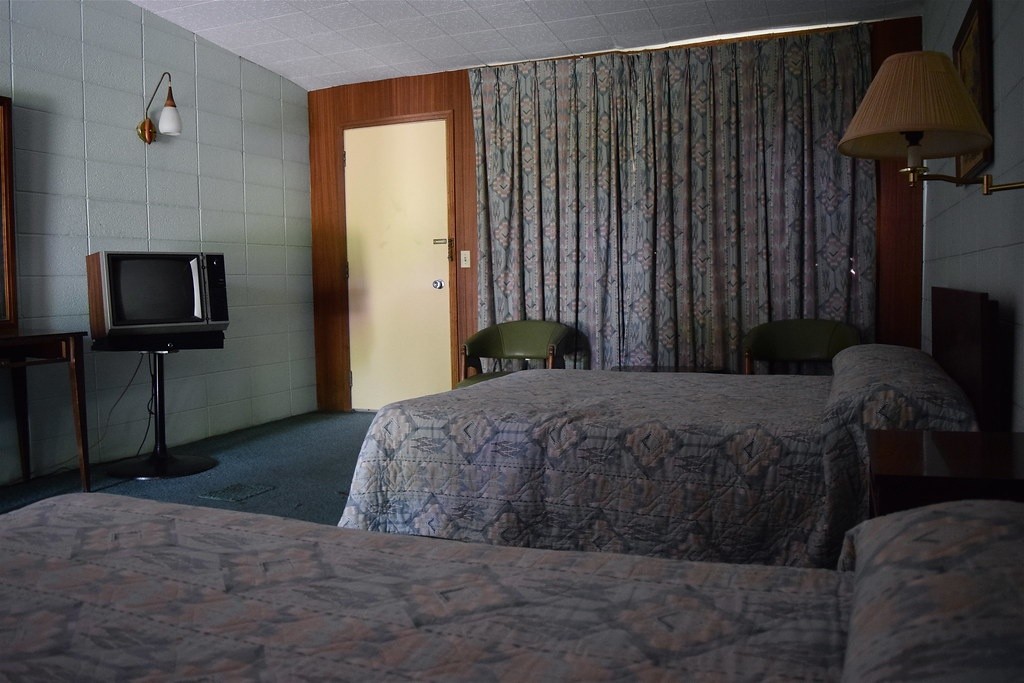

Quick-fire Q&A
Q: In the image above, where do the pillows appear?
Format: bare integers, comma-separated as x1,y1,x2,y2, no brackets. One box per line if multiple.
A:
838,497,1024,683
826,342,985,443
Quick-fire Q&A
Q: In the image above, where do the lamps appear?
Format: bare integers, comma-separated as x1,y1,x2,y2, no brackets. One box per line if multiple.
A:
135,71,183,145
836,50,1024,195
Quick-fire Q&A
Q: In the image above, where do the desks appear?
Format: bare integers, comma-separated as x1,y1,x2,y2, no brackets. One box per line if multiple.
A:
0,331,90,495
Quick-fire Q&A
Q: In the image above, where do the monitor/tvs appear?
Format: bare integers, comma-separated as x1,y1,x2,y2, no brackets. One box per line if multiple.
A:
85,249,230,339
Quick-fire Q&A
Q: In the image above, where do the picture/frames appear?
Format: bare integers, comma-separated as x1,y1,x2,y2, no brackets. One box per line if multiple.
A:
950,0,995,186
0,95,20,333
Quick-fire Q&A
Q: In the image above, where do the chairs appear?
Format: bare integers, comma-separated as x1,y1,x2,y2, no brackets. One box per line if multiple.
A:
455,320,568,389
742,320,861,375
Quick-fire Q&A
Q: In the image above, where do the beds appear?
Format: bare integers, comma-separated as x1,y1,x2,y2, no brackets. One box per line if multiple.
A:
336,287,1000,569
0,499,1024,683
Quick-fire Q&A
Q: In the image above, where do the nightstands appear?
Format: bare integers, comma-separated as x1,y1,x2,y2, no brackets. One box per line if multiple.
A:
866,431,1024,519
